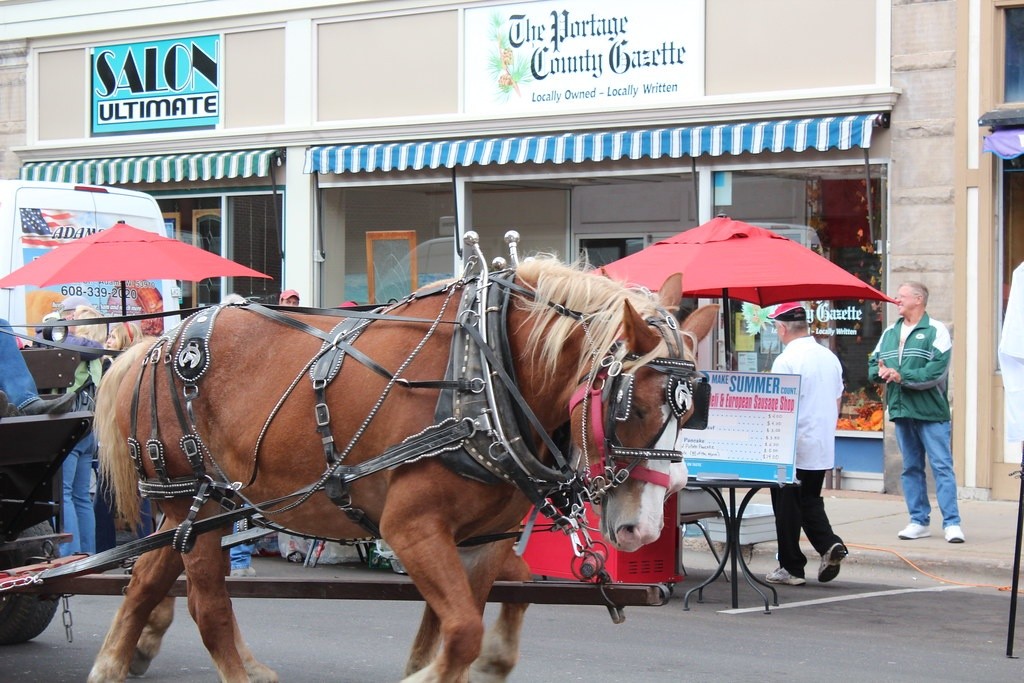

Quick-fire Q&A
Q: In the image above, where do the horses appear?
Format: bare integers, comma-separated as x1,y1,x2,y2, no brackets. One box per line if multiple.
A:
86,256,722,683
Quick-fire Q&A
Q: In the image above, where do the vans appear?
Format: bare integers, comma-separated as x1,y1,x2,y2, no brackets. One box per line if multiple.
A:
0,179,180,347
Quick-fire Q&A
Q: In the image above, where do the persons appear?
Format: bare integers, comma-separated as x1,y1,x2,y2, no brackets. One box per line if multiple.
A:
33,296,256,576
765,302,849,586
867,280,965,543
279,290,299,307
0,319,76,416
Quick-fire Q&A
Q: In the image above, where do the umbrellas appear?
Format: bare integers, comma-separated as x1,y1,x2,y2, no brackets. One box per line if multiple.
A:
587,214,901,371
0,220,274,324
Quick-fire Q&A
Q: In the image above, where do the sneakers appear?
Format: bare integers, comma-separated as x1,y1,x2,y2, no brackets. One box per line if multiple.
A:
818,542,844,582
945,526,965,542
766,566,806,586
897,521,930,539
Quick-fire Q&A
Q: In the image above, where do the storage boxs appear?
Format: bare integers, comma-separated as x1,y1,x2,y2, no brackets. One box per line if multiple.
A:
678,486,722,513
707,501,776,547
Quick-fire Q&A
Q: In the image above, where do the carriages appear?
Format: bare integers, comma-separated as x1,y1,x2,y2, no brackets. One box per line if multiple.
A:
0,216,704,683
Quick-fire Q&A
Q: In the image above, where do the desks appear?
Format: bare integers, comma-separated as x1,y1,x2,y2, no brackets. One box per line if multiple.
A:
679,512,727,583
669,478,790,616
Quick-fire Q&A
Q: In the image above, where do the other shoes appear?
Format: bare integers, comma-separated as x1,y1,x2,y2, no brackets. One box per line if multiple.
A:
19,391,76,416
231,566,256,578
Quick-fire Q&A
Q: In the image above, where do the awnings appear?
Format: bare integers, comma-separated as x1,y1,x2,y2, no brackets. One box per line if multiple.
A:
303,111,891,175
18,147,287,186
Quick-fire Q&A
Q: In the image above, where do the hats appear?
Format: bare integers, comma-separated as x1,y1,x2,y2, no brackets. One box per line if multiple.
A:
280,290,299,300
767,301,806,320
60,295,92,312
341,301,357,306
